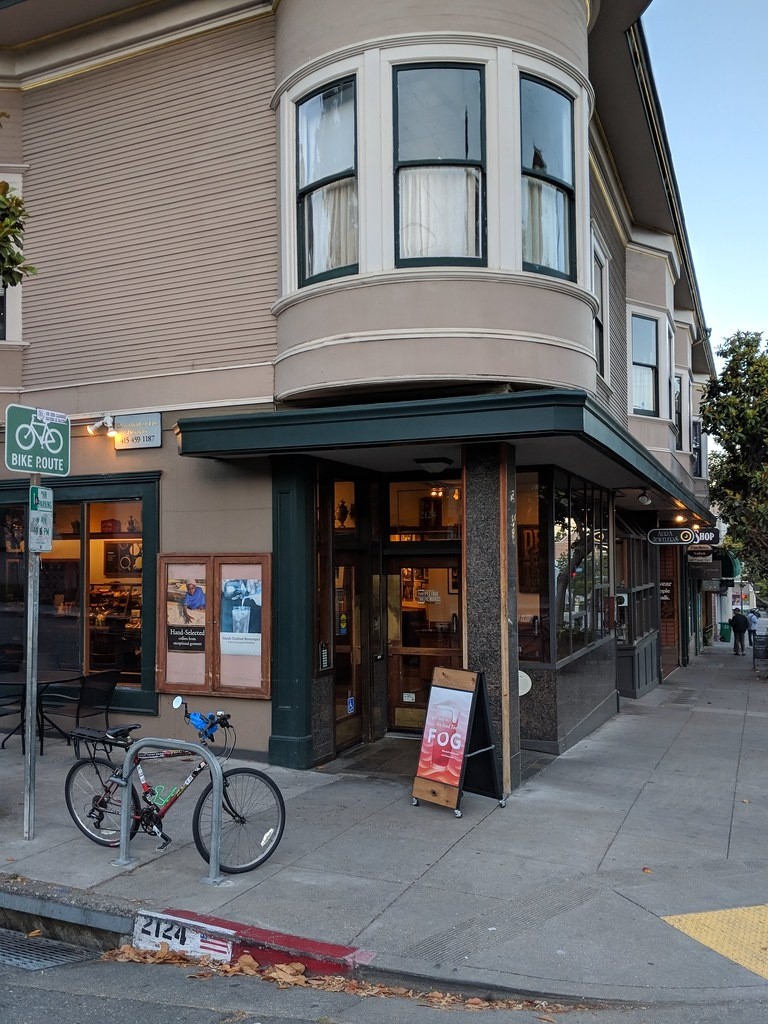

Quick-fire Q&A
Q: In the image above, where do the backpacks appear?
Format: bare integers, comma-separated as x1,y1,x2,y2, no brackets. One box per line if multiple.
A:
748,615,755,628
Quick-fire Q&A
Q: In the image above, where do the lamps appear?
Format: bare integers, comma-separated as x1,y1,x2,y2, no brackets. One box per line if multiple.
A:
430,486,445,498
86,420,104,434
673,514,684,525
105,419,117,437
691,523,700,531
638,490,649,504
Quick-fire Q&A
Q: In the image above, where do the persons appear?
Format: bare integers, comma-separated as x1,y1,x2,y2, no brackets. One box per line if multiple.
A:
106,581,127,614
728,608,749,655
747,608,759,646
182,578,205,624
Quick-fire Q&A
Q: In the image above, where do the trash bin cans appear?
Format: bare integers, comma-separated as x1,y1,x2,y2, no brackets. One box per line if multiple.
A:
717,621,732,643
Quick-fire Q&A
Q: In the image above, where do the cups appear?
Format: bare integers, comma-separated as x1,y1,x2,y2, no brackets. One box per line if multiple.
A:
232,606,250,633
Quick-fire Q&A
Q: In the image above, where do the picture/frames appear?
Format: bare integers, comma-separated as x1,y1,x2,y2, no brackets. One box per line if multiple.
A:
154,551,272,699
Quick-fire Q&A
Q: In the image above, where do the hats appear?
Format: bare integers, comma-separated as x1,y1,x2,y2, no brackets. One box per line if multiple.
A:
186,579,196,587
732,608,740,612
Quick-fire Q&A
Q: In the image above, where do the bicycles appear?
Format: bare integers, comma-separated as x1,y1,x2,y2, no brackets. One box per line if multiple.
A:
62,696,286,874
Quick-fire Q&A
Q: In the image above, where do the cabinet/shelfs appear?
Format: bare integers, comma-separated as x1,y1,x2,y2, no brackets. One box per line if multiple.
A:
0,603,142,682
412,622,542,683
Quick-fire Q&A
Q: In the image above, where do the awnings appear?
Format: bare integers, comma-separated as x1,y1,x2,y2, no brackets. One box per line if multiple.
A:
713,549,740,578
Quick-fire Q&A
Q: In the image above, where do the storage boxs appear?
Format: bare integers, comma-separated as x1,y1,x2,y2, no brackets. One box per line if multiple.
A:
100,519,122,533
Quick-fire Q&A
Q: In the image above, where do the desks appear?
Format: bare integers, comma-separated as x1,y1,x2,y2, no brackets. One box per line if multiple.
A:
0,670,84,756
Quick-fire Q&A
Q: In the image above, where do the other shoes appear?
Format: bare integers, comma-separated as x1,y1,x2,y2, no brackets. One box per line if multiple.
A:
742,652,746,655
733,653,740,656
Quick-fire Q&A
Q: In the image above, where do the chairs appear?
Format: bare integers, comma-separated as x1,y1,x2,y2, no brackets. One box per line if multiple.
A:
41,669,122,760
0,694,26,755
